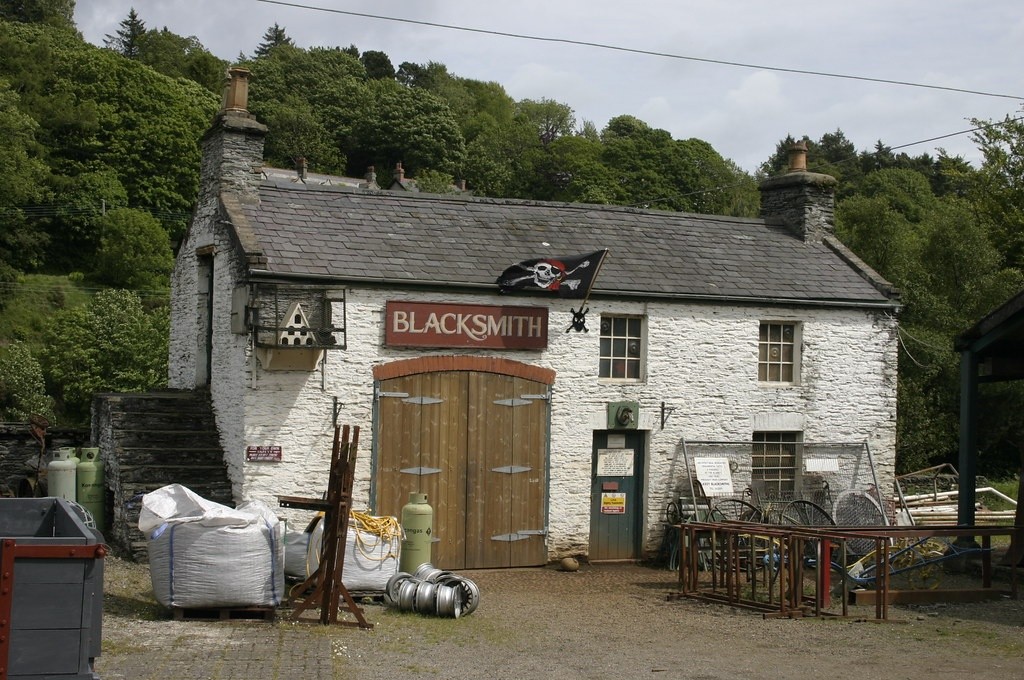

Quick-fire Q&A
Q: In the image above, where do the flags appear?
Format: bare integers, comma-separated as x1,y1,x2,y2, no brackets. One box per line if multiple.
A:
495,249,606,300
